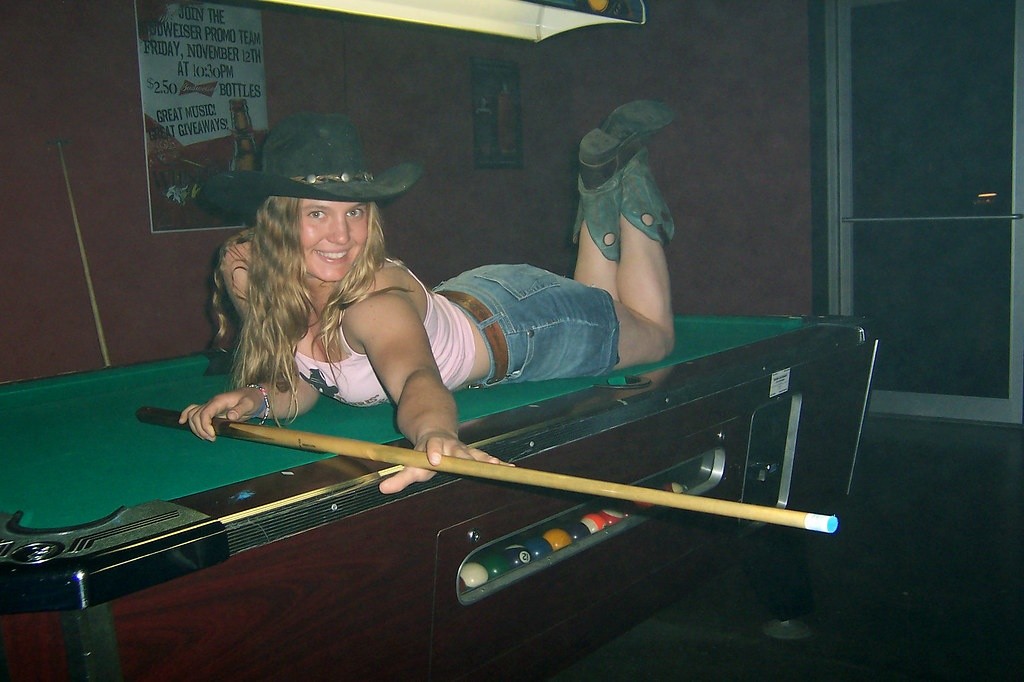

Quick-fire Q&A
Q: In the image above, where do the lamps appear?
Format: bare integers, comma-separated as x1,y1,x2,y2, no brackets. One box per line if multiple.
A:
270,0,647,43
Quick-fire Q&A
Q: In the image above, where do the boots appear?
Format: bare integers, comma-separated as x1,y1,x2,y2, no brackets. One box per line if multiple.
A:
572,97,675,262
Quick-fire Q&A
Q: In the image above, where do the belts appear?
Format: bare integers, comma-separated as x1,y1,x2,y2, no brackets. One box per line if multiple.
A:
436,290,509,384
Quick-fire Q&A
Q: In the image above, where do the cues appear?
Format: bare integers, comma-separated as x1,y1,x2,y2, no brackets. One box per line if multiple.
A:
133,405,841,536
55,139,113,368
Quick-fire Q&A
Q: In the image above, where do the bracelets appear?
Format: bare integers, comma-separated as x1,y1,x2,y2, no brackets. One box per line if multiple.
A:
246,384,270,427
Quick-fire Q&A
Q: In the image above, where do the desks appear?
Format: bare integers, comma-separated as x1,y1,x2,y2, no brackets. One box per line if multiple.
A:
0,313,887,682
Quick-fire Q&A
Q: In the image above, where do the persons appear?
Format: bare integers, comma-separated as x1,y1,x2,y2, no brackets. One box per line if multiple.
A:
178,98,679,494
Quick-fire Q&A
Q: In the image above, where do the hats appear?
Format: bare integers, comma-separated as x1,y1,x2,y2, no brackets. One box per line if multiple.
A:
198,111,426,227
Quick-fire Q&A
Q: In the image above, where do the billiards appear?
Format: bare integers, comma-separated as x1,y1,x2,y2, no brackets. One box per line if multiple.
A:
459,481,685,592
